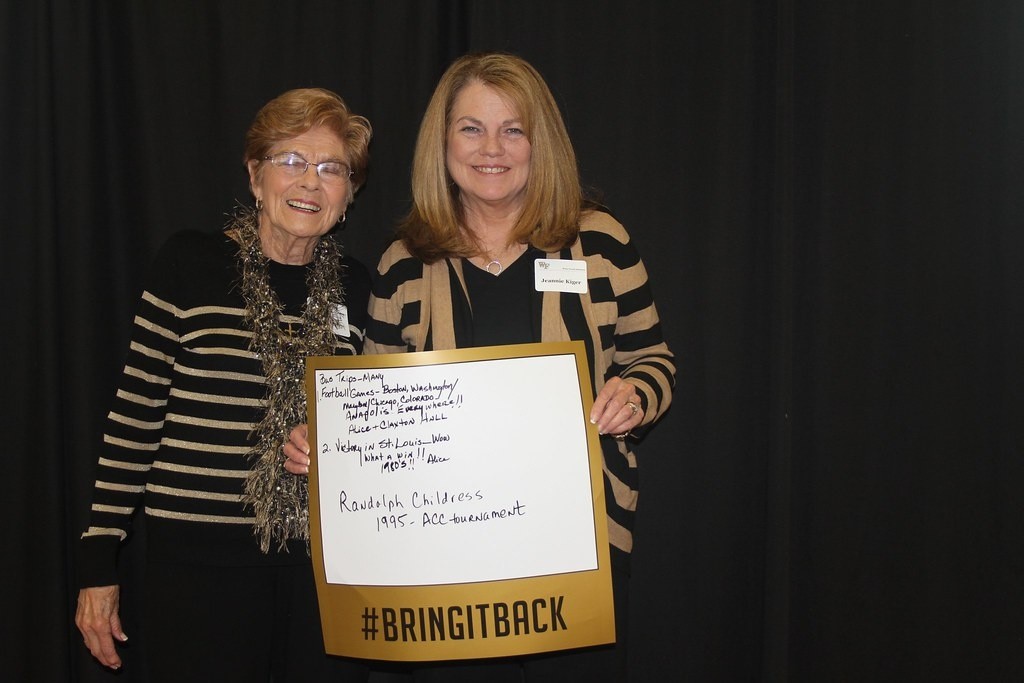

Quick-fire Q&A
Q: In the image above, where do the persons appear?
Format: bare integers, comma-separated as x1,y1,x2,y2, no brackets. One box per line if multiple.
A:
75,89,372,683
283,53,675,683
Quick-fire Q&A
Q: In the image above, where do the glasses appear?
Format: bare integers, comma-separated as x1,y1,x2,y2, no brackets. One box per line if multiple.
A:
259,152,354,186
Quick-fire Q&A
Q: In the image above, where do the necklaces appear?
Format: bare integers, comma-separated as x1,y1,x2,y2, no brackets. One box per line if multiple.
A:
487,245,508,276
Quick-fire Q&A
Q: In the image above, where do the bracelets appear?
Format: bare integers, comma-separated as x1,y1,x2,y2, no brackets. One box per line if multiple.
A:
612,429,632,438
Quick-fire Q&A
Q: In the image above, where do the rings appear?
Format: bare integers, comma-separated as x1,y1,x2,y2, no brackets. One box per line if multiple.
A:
625,402,639,416
